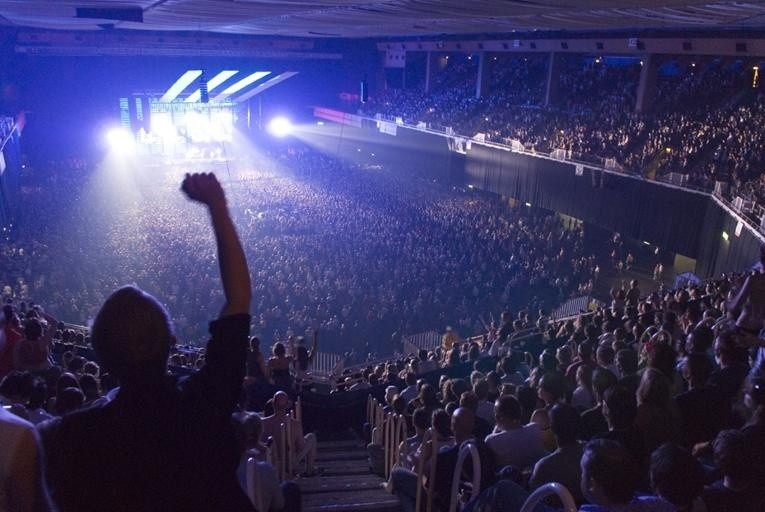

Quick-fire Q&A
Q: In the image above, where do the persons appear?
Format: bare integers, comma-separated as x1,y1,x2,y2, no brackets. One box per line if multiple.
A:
1,56,764,512
